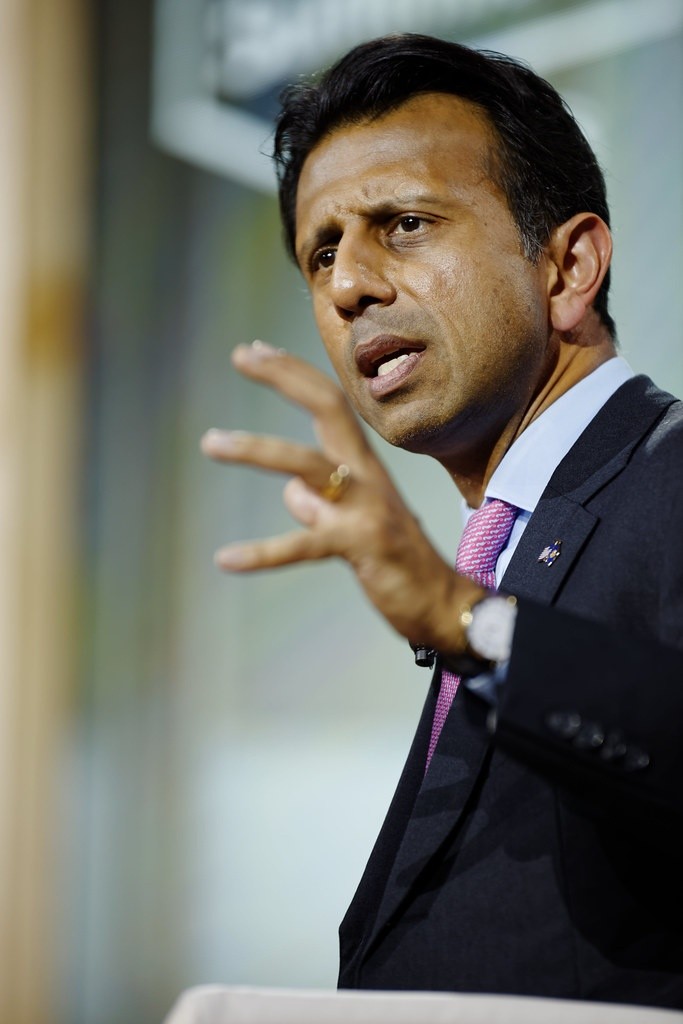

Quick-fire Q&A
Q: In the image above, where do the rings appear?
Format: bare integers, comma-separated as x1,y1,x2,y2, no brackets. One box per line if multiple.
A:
320,466,351,503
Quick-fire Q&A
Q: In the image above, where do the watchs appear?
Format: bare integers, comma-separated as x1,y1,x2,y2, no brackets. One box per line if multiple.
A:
442,592,517,678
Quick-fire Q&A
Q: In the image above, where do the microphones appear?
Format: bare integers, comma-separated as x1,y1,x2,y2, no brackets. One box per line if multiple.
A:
407,638,439,668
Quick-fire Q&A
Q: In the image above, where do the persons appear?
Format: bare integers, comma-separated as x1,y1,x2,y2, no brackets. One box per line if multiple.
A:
201,33,683,1014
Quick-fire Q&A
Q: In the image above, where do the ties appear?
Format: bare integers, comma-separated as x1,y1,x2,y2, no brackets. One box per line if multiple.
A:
426,498,519,782
